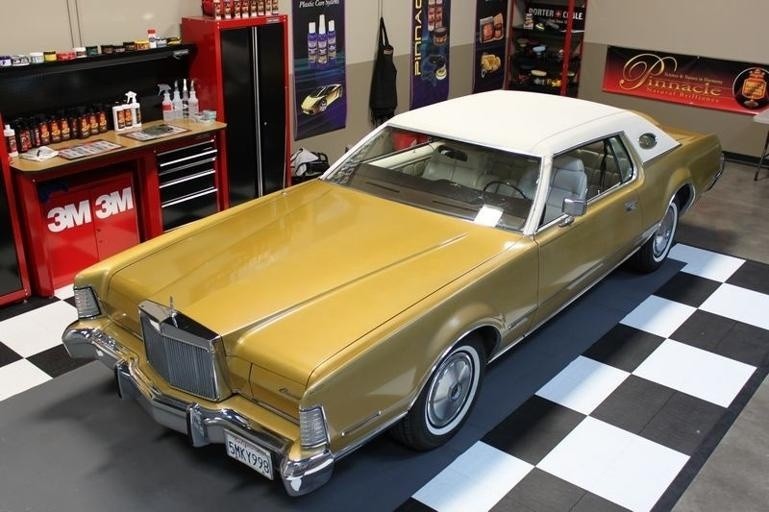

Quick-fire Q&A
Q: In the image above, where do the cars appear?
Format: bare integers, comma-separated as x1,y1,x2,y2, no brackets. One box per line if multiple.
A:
61,87,726,502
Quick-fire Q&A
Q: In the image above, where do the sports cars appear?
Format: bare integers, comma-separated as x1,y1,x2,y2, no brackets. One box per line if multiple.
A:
300,83,344,116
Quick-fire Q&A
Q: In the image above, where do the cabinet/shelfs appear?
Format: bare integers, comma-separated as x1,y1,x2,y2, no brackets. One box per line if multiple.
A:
505,0,587,97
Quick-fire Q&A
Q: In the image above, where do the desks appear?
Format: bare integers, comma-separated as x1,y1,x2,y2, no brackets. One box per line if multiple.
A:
11,115,226,298
752,106,769,181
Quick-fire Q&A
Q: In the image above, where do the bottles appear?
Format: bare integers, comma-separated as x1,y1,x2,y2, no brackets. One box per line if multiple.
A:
3,100,133,158
147,28,156,49
307,14,336,71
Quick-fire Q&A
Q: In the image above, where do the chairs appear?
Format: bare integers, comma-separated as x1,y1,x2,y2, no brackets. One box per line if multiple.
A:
571,142,630,202
489,150,528,184
421,141,487,193
513,156,588,230
